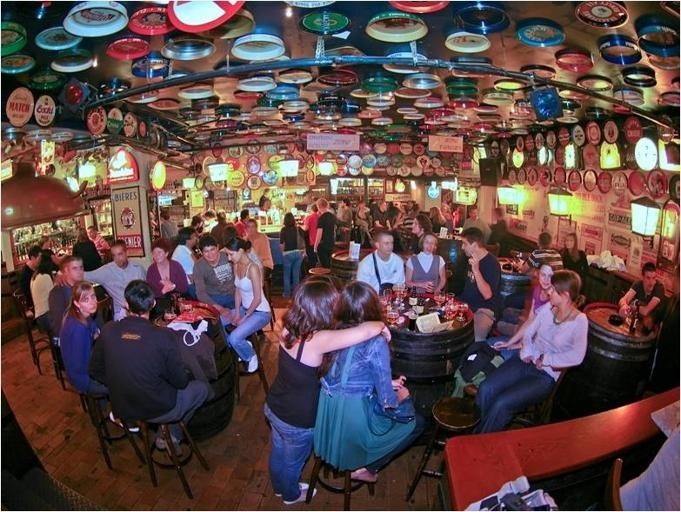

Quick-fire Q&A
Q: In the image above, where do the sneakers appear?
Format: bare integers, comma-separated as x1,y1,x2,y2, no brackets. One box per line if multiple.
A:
109,411,140,433
351,467,376,482
247,353,258,373
156,434,178,449
238,340,253,362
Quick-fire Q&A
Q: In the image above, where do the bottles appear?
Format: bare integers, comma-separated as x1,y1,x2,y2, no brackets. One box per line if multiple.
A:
408,308,418,333
337,180,359,194
93,201,112,235
626,302,640,333
16,224,77,261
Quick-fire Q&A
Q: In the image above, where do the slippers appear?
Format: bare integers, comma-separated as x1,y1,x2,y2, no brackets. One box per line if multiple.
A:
275,482,317,505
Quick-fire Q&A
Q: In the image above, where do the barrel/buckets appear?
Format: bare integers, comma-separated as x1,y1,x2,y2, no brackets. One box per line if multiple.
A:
495,256,532,321
330,248,372,291
384,297,475,445
435,232,469,296
550,300,653,425
152,303,237,446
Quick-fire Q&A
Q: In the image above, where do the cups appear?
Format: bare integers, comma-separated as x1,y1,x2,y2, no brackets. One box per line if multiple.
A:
165,293,193,325
512,263,519,272
255,210,266,231
440,227,449,239
383,283,468,327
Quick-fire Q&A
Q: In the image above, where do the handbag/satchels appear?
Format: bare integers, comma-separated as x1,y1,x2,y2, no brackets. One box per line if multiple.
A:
373,390,416,424
153,290,185,314
296,236,305,249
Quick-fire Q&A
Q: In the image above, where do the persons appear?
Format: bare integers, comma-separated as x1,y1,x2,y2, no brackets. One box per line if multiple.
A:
251,274,392,505
618,425,680,511
22,188,679,451
312,280,428,484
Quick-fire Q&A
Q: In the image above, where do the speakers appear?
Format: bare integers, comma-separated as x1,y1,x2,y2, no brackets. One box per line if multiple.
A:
479,158,498,186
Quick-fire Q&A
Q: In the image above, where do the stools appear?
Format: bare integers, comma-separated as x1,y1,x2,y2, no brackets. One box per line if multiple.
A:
13,215,670,509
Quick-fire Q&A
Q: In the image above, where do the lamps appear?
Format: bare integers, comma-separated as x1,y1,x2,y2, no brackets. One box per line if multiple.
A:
205,156,230,182
315,160,336,178
494,179,668,240
276,158,301,181
